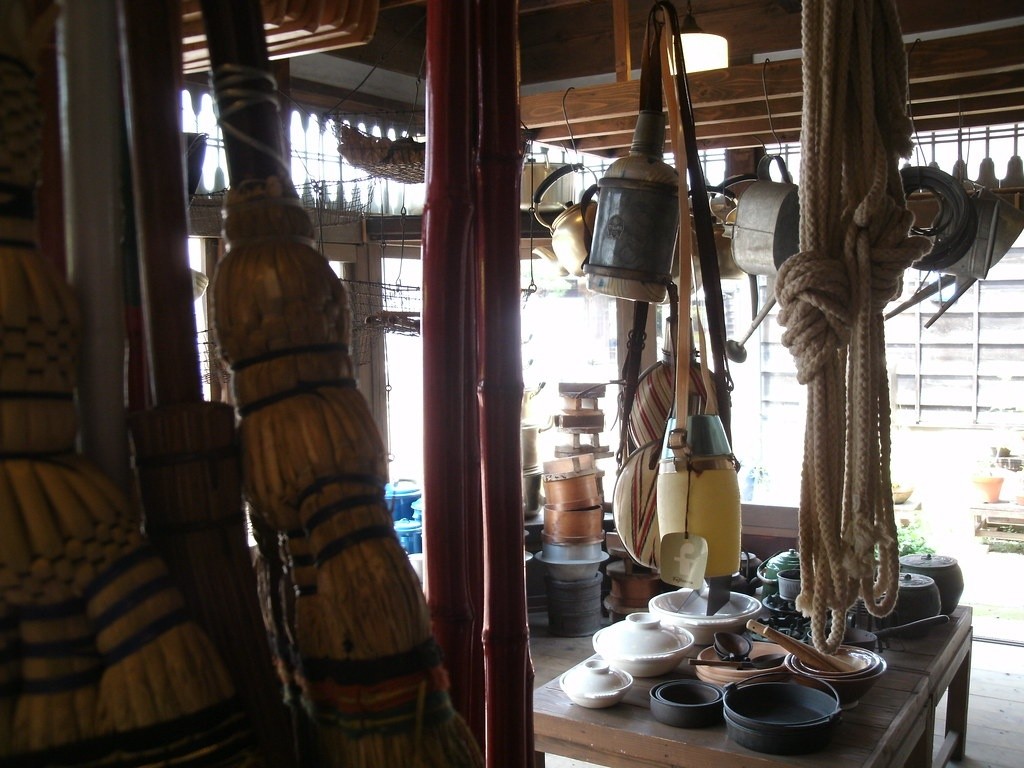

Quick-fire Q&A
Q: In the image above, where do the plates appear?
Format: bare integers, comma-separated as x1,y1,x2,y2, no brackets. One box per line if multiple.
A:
695,642,789,686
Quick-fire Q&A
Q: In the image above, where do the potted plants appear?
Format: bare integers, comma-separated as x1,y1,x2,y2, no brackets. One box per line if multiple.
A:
893,482,913,503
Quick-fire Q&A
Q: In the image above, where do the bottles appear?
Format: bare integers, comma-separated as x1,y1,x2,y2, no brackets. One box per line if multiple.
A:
656,415,744,580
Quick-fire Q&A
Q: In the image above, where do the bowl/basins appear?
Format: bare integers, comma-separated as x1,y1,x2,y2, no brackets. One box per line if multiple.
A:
649,678,724,727
648,587,762,645
784,644,887,698
723,672,843,754
558,659,636,707
592,612,695,677
807,626,877,651
892,490,912,504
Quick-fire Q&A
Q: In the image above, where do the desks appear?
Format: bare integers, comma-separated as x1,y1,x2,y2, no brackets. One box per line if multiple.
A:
533,580,975,768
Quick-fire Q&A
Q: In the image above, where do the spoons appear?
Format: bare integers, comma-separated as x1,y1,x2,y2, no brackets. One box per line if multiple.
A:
688,654,786,670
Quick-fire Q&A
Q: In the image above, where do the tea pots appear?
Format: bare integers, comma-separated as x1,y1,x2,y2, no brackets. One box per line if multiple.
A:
756,548,803,602
740,546,764,587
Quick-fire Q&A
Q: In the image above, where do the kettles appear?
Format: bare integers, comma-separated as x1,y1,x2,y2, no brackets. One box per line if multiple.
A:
531,163,600,277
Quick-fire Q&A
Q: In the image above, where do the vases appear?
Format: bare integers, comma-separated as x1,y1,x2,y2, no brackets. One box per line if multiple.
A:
974,477,1005,502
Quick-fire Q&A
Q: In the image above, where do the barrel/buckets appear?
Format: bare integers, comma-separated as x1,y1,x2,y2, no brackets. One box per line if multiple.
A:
385,479,422,521
395,518,422,554
410,497,422,521
971,476,1005,504
542,571,604,637
